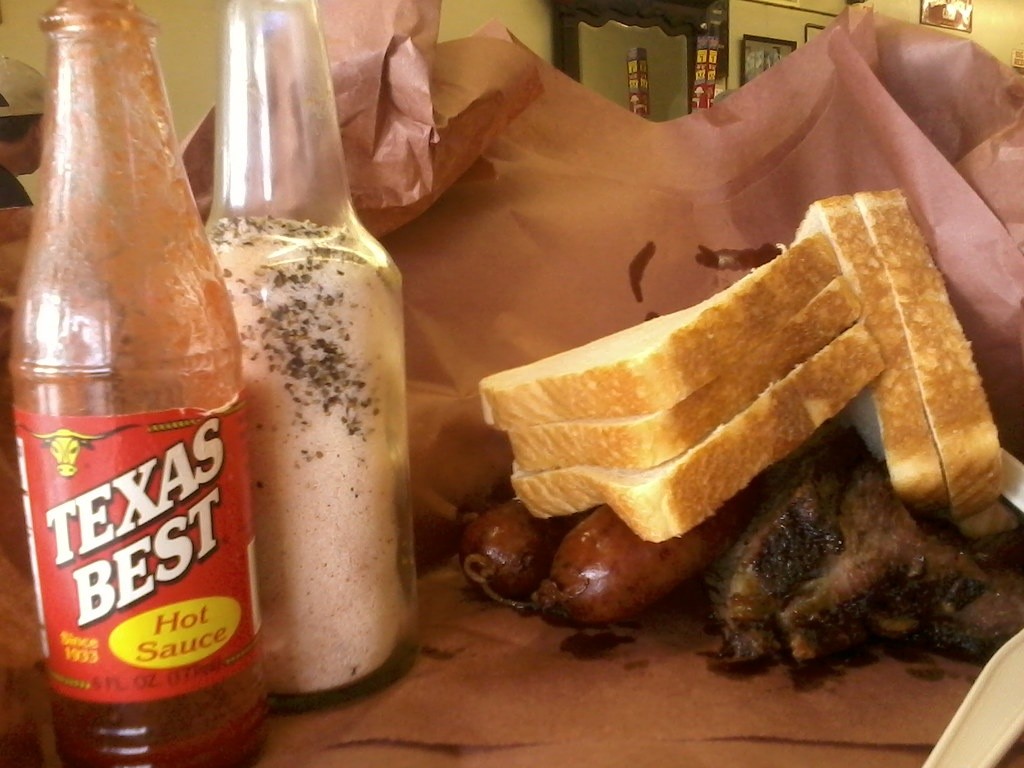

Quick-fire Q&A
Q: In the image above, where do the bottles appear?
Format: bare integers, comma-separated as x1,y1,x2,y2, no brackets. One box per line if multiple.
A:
6,0,272,768
203,0,419,705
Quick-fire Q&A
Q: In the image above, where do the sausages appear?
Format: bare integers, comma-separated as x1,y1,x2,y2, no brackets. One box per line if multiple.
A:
450,460,767,629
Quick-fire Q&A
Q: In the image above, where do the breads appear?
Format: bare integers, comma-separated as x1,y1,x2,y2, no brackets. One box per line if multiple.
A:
477,177,1002,543
